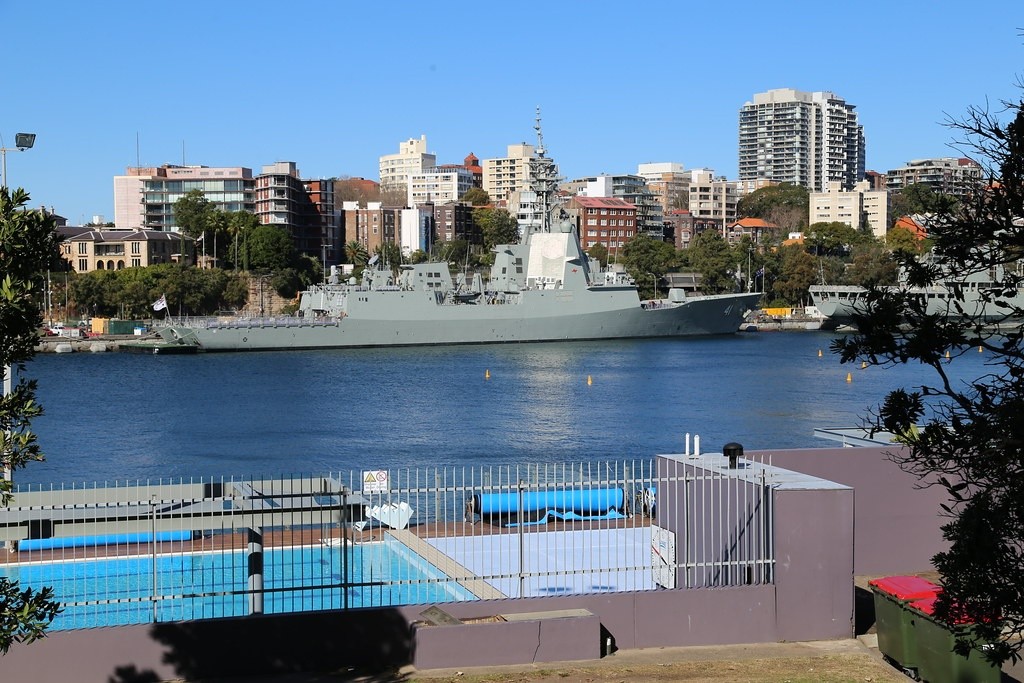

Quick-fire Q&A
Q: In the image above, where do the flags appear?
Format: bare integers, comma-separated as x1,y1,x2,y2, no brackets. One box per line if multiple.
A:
151,296,167,311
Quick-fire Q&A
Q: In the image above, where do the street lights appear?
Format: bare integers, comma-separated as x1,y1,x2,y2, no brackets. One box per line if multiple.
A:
648,273,656,300
65,272,68,323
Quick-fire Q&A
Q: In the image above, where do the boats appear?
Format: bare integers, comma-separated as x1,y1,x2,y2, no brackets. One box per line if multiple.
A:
808,245,1024,336
150,105,766,352
454,291,481,300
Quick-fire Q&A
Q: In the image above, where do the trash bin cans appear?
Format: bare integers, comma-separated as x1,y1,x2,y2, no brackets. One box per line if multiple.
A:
904,594,1002,683
867,575,944,681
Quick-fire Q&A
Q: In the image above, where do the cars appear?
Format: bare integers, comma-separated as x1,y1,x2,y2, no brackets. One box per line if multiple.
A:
78,319,92,327
41,326,66,336
